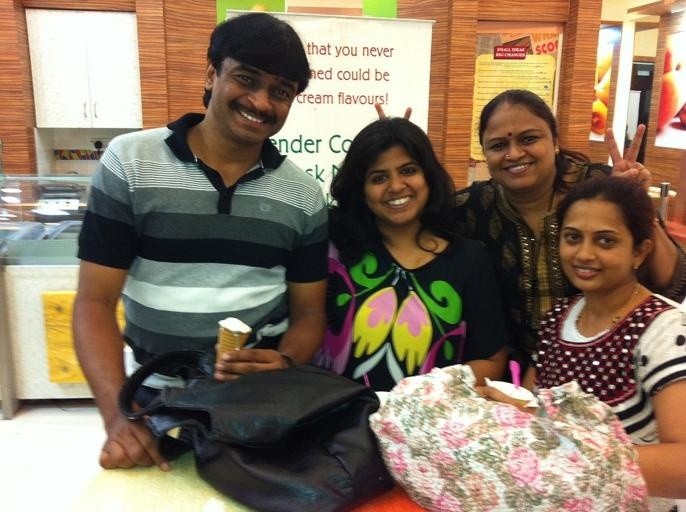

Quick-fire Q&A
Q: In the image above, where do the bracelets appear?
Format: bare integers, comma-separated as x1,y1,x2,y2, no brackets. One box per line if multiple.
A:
281,354,295,368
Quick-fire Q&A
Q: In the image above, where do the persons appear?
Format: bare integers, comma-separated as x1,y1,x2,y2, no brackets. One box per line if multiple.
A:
474,177,686,499
308,118,509,388
375,90,686,363
72,13,330,471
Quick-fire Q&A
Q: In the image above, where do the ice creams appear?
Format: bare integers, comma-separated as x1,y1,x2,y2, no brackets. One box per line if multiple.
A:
216,316,253,363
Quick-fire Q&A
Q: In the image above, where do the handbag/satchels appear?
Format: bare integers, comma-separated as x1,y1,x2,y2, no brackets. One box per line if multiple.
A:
368,360,648,511
118,348,392,511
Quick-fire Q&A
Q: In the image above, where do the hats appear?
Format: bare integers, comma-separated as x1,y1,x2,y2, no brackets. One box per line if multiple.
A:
207,13,311,96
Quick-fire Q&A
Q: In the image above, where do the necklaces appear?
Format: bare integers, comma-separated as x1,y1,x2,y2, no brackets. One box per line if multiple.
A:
576,283,641,339
